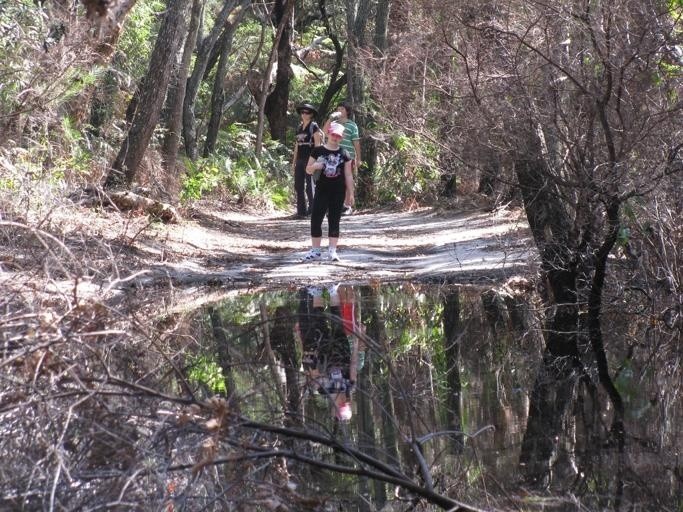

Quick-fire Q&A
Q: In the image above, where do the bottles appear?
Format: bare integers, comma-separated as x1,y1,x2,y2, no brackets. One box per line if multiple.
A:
327,110,345,122
310,155,327,181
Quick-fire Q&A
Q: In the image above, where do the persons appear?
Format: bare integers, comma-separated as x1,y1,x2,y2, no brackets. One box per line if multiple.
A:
300,122,355,262
270,283,366,421
290,104,320,218
323,102,361,216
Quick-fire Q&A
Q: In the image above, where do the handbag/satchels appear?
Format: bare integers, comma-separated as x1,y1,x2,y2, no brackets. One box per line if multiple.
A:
313,163,326,184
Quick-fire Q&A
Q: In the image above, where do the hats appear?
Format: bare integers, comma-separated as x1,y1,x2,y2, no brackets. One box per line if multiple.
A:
335,402,352,421
296,104,319,117
328,122,345,136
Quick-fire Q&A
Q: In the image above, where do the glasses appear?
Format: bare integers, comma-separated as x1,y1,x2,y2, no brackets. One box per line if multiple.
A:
301,111,311,114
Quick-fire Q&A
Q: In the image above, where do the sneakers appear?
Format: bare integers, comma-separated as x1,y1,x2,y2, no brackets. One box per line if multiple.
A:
327,281,341,293
306,284,324,296
327,249,339,261
341,207,352,215
302,249,322,262
288,212,312,220
